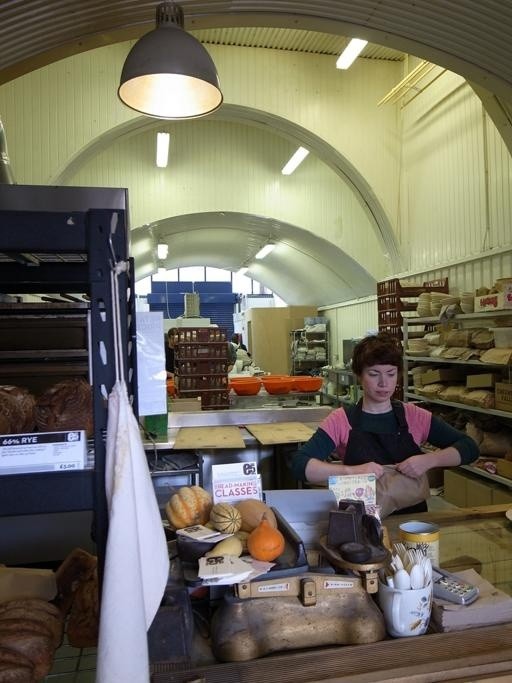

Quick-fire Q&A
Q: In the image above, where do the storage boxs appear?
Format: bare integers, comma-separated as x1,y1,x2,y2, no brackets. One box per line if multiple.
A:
443,468,511,509
486,324,512,413
473,291,512,312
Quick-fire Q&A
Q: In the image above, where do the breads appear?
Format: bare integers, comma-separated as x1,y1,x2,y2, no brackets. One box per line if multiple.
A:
34,376,94,436
0,385,38,435
0,547,99,683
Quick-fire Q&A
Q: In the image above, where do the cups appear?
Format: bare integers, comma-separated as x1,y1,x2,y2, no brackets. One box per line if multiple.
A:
378,582,434,637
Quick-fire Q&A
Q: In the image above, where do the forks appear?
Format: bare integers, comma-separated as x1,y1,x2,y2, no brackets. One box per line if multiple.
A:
394,541,429,564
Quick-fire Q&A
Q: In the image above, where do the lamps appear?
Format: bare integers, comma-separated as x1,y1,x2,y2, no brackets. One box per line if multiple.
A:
118,0,223,120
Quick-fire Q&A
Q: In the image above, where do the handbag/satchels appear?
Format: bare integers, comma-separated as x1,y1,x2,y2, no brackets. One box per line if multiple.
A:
363,461,433,523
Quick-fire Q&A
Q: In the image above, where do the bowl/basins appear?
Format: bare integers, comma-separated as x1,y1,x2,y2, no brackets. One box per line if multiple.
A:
231,375,323,396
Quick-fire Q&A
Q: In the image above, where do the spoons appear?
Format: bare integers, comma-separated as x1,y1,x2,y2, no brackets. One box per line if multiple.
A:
394,557,433,590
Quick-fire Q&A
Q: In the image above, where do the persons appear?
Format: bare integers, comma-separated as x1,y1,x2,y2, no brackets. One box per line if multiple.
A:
227,342,238,372
232,332,247,352
165,325,177,380
290,332,480,516
230,341,251,366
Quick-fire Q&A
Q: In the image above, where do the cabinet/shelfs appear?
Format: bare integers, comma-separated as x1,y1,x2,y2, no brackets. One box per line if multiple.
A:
289,331,328,375
403,309,512,488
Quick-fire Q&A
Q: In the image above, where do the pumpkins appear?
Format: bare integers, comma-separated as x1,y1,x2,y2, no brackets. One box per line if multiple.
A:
165,484,286,561
